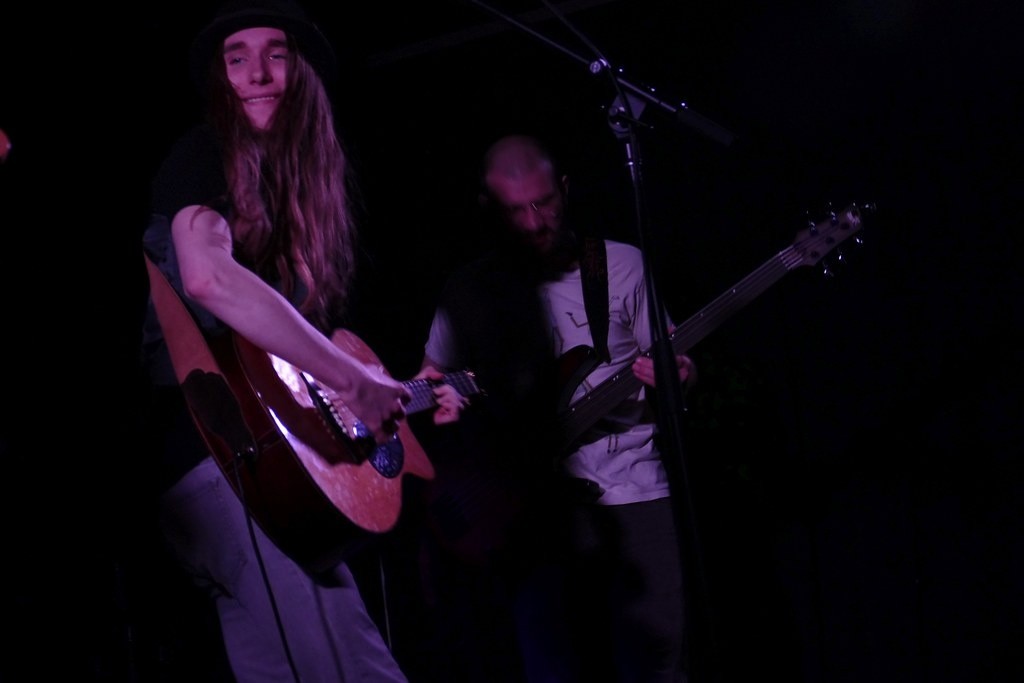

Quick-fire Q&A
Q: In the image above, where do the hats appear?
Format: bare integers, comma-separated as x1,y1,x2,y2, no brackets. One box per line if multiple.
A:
187,0,335,112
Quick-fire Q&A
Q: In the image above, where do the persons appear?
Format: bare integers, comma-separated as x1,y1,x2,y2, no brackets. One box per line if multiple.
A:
127,11,470,683
414,132,696,683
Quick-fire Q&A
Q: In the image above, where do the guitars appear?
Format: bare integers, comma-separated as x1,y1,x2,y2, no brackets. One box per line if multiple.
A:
422,201,875,561
223,325,489,557
